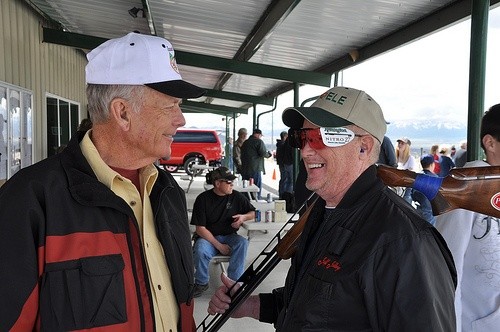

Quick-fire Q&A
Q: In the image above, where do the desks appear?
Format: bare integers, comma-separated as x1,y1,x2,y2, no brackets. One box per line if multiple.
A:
240,202,299,243
203,180,260,200
187,165,218,193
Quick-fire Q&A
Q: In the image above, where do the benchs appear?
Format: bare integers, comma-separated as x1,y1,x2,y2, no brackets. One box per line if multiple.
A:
187,199,195,212
180,175,190,181
211,255,230,275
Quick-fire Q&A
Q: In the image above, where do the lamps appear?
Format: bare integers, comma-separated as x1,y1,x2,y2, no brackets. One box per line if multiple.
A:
128,7,146,18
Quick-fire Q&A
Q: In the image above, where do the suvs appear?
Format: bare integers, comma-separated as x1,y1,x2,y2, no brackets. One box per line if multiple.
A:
157,129,222,177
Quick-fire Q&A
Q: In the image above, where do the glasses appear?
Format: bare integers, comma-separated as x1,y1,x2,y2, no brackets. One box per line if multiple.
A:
288,127,363,149
219,179,233,184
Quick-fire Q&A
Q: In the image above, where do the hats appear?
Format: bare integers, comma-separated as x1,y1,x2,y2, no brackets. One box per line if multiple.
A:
253,129,263,136
212,167,237,181
282,86,387,144
421,154,440,164
397,137,411,145
85,31,207,100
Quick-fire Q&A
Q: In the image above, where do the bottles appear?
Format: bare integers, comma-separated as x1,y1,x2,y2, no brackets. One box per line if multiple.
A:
195,158,198,166
206,160,209,167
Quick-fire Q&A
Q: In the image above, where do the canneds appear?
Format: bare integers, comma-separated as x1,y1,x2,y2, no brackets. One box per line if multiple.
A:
254,209,261,222
243,180,247,188
265,210,272,223
267,193,272,203
249,178,253,186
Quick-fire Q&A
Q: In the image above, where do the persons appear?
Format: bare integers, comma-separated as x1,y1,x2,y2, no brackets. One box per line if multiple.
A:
187,127,468,298
208,86,458,332
0,32,209,332
430,103,500,332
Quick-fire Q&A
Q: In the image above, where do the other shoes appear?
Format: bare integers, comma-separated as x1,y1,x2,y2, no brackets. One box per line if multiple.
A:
193,283,209,298
